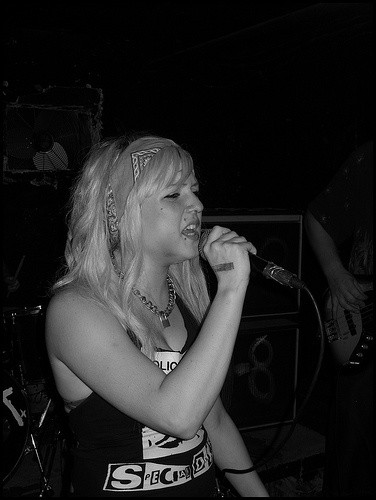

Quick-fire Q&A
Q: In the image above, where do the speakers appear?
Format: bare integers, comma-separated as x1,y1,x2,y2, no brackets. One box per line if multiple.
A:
218,317,301,434
196,208,304,319
0,306,66,416
0,170,82,306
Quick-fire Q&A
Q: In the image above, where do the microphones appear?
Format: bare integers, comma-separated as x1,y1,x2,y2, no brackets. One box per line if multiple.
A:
198,228,306,292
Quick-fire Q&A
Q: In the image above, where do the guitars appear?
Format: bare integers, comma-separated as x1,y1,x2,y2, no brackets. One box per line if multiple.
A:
313,278,376,372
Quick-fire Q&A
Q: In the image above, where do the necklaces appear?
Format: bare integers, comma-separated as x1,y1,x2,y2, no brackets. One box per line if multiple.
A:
110,257,177,328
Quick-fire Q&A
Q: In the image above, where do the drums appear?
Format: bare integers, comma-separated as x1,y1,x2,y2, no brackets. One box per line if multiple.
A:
0,365,33,487
0,302,55,385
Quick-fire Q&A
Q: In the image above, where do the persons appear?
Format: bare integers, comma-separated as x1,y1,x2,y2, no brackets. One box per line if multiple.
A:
0,248,20,308
45,134,272,497
298,137,376,499
